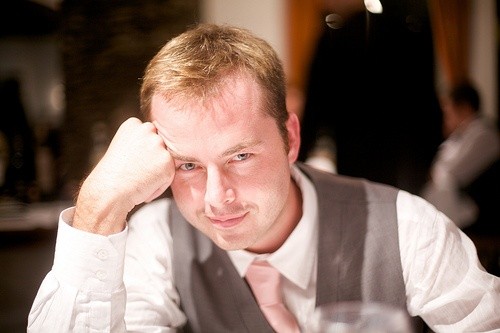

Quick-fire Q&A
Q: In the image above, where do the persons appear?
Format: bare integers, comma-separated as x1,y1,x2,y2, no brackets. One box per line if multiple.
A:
297,0,448,196
419,73,500,278
26,22,500,333
